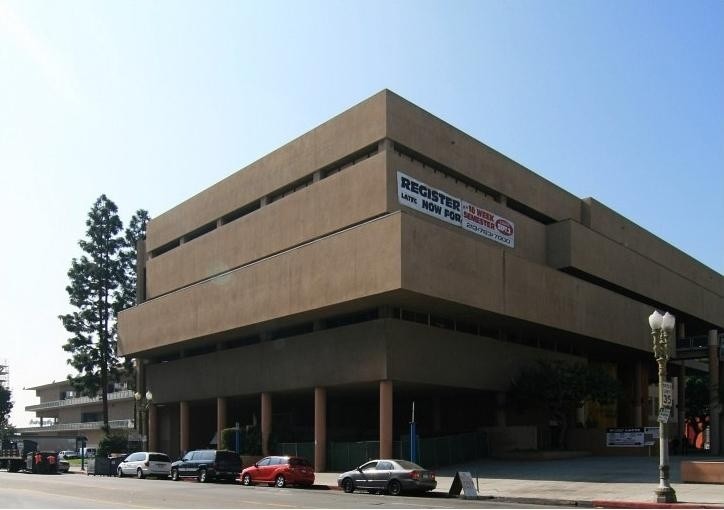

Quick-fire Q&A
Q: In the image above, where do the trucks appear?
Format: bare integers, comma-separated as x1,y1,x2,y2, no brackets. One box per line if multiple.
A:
0,439,25,472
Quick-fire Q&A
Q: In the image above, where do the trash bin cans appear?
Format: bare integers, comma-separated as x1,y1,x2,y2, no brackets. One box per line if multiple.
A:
86,448,128,477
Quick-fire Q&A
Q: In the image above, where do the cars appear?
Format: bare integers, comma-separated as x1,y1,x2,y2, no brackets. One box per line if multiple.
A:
337,459,437,496
57,447,98,472
116,449,315,487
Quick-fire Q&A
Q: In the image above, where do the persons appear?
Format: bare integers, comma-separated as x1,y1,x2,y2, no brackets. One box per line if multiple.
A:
34,452,42,474
47,453,57,474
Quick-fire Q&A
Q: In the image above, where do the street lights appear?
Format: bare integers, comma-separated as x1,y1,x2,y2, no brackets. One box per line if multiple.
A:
648,308,674,494
134,390,153,451
